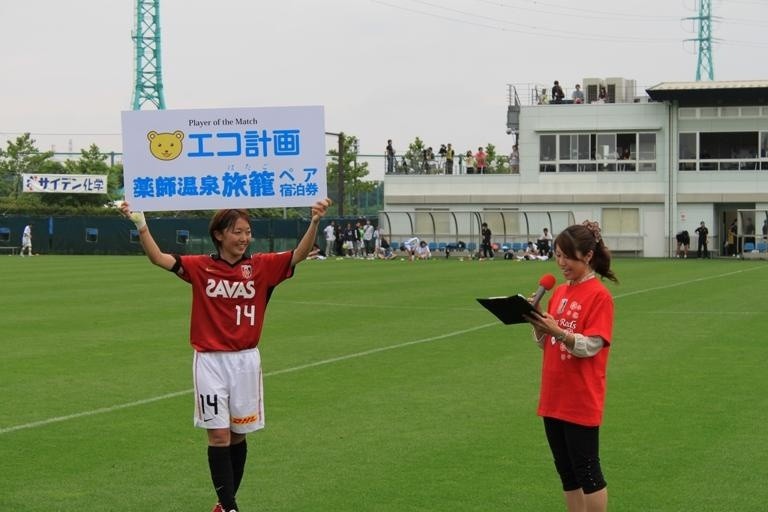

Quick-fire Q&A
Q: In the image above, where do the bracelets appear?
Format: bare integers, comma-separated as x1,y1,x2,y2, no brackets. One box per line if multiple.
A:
556,329,569,342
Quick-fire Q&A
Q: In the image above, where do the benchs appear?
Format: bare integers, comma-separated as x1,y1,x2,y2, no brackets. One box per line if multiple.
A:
0,246,18,255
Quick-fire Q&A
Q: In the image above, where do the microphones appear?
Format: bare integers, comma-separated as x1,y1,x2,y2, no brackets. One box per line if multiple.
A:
529,274,556,309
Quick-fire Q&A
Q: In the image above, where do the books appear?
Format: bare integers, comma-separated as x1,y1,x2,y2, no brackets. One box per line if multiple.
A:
475,293,542,325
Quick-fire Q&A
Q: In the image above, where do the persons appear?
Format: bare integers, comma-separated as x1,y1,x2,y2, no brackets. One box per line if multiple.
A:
323,219,392,260
505,145,519,173
385,138,394,173
674,230,690,258
398,237,419,262
517,254,536,261
19,222,34,258
724,216,767,258
120,197,333,511
527,219,621,512
479,222,494,260
694,220,709,259
420,142,487,173
537,89,547,103
551,80,564,103
571,84,583,104
516,251,553,262
515,241,542,262
538,227,553,256
305,243,327,261
597,86,608,103
414,240,431,259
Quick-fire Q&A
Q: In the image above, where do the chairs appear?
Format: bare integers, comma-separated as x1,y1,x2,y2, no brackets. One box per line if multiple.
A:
744,242,767,252
390,241,529,257
